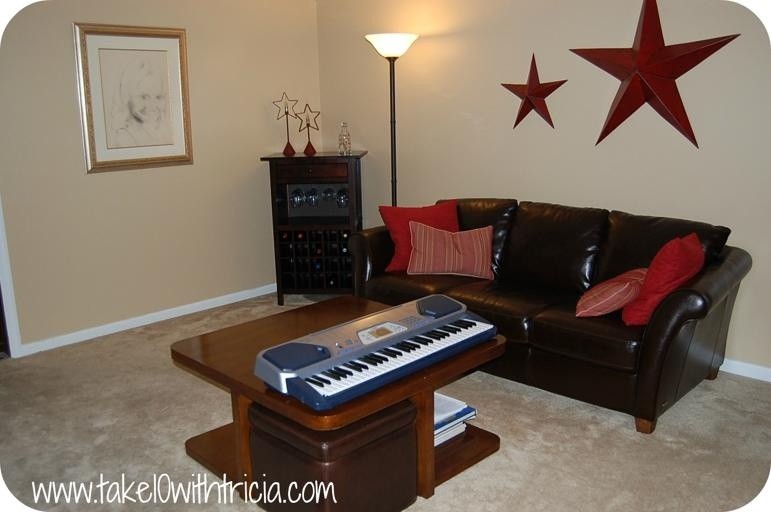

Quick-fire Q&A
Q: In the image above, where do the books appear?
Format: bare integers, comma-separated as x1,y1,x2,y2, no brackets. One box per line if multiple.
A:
433,392,477,448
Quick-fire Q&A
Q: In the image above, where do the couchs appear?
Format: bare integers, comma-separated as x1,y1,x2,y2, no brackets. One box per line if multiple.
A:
347,198,752,434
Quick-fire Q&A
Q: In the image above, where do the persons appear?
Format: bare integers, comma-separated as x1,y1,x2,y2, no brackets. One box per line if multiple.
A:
110,60,165,147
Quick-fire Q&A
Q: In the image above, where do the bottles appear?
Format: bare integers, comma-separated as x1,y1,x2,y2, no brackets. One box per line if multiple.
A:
338,121,352,154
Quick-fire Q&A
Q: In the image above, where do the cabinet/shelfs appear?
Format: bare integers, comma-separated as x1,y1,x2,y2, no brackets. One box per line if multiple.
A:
261,151,368,306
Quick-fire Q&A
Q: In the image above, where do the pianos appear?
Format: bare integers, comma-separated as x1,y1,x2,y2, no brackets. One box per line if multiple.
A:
254,294,497,411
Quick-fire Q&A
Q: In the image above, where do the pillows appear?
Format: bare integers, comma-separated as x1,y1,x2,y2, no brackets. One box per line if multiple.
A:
407,221,494,280
622,233,705,326
379,199,460,274
576,268,648,318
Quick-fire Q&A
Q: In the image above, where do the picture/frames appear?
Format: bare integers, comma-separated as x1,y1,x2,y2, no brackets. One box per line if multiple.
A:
72,22,193,174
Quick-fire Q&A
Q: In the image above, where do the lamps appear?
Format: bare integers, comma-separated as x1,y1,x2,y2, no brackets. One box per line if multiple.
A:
364,33,418,207
